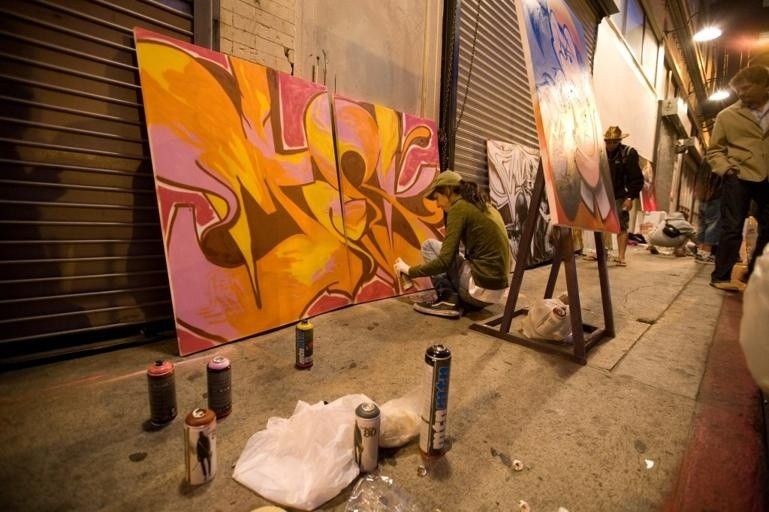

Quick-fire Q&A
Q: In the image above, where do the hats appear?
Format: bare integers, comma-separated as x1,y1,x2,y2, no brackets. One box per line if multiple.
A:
603,126,629,140
424,170,463,201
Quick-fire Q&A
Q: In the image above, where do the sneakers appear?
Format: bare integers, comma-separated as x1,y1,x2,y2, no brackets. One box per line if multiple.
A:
413,291,463,318
695,252,717,263
709,280,739,292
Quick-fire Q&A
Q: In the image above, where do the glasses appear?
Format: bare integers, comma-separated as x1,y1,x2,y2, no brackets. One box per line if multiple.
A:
606,141,620,144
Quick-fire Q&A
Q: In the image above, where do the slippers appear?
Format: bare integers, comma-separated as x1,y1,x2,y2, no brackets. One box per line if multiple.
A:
582,255,598,261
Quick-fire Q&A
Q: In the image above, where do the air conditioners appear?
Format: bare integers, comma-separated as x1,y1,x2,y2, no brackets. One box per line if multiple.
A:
662,99,705,166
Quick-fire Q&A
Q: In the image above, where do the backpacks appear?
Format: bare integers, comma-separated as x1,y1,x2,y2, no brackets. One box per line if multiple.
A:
695,156,721,203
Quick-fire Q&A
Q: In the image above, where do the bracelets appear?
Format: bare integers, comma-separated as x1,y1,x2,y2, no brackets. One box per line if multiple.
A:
626,197,632,200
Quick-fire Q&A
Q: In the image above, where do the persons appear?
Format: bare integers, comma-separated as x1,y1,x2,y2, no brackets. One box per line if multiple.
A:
583,125,644,265
694,157,727,264
706,65,768,292
646,211,697,256
392,170,514,318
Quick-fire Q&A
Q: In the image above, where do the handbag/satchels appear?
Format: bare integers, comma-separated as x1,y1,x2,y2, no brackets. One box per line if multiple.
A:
662,223,681,237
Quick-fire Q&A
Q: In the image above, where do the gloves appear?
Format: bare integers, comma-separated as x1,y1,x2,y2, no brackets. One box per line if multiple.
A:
393,257,411,279
